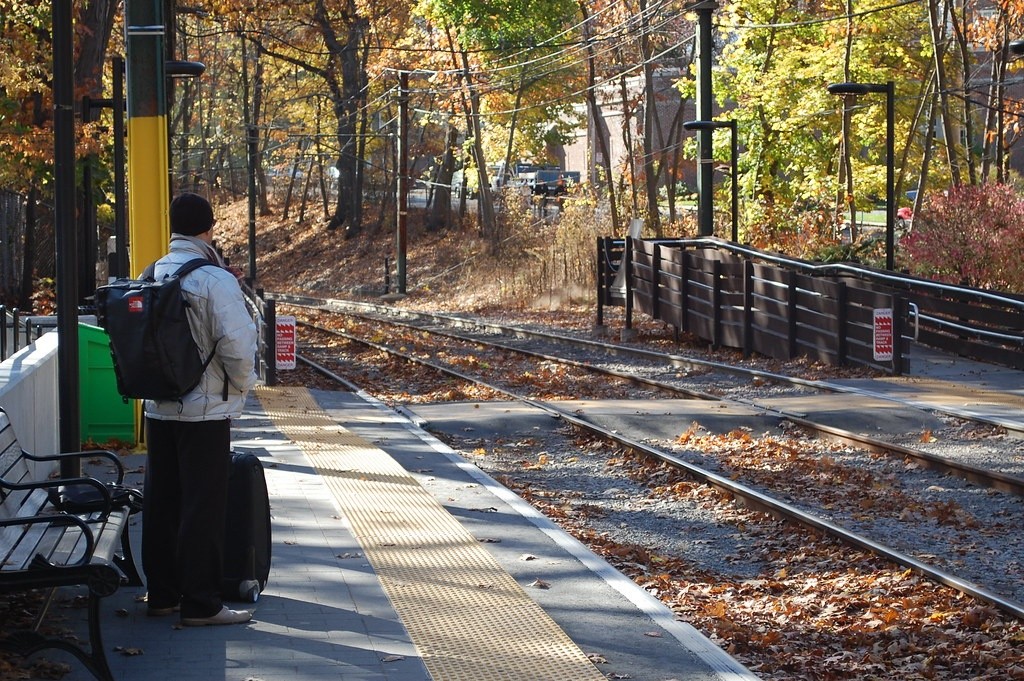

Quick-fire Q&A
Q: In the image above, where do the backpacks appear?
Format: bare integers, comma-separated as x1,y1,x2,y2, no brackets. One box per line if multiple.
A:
95,257,226,405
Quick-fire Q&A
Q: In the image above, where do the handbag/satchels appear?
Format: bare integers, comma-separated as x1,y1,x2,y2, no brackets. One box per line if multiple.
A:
48,481,145,515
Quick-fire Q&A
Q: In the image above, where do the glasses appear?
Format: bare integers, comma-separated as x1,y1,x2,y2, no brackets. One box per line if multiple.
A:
211,218,216,226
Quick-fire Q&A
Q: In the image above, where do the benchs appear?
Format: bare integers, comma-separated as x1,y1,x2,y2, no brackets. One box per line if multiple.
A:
0,405,147,681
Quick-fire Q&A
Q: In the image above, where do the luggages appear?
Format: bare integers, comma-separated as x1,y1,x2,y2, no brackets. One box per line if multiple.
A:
223,451,272,605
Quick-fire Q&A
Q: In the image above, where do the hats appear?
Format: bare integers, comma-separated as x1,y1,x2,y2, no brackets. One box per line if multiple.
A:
169,192,213,236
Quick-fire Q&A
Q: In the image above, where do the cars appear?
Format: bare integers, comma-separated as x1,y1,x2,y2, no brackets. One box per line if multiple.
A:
451,165,503,200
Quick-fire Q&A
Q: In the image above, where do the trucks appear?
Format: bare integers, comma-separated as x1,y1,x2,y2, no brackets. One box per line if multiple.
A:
492,159,581,208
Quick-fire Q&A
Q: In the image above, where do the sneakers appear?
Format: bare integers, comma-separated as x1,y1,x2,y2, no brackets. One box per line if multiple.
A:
181,604,250,625
148,603,182,616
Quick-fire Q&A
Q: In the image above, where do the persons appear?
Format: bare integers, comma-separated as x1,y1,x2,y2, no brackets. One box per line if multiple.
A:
133,194,259,626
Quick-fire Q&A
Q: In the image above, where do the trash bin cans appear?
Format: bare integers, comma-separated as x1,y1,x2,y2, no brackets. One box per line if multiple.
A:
79,322,143,446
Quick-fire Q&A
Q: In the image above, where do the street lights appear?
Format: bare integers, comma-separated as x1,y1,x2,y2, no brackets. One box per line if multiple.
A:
682,118,738,244
112,53,205,284
826,81,896,270
